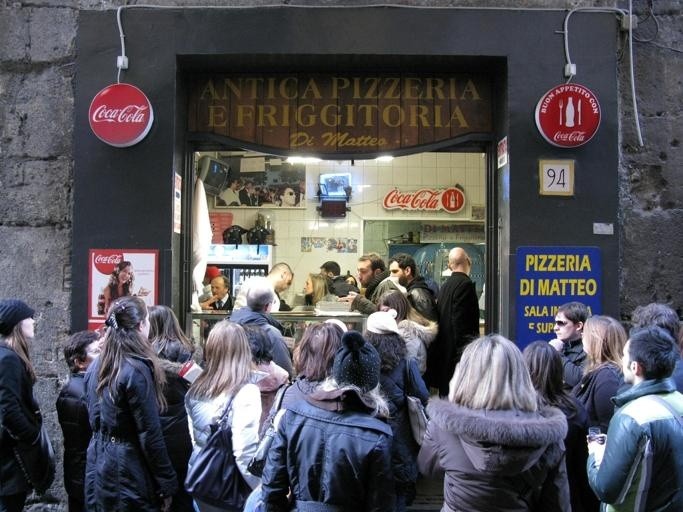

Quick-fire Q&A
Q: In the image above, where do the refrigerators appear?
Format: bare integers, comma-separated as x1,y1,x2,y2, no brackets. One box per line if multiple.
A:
202,244,231,308
231,244,272,307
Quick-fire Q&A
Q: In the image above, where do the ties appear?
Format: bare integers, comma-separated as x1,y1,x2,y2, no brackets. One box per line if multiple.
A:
218,300,222,309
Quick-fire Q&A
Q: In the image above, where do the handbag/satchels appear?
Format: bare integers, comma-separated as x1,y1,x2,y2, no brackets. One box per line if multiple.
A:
405,354,428,446
248,384,290,476
185,381,253,511
601,435,653,511
15,423,56,497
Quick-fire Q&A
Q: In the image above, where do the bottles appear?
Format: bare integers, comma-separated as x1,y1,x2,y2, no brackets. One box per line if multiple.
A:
236,268,264,284
97,287,104,314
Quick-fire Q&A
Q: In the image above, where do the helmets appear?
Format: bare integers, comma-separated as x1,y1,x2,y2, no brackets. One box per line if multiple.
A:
222,225,247,245
246,225,270,244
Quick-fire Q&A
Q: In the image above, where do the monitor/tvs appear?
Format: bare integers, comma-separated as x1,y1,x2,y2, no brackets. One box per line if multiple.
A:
198,155,230,197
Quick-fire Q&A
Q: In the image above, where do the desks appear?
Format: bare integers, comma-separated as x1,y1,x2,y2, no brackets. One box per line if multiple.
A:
186,305,368,338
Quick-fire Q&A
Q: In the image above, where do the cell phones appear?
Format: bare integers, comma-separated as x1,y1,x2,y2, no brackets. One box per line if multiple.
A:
589,427,601,443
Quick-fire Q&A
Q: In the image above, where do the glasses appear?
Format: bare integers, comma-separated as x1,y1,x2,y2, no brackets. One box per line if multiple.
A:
286,192,296,196
578,330,584,340
554,320,566,327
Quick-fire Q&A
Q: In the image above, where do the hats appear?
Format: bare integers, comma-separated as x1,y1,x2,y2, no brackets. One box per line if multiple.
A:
366,308,399,335
0,299,35,336
208,266,221,280
333,330,380,392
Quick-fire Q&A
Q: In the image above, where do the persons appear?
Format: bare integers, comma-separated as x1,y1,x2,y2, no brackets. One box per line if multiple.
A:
199,274,235,310
100,261,152,317
436,245,480,394
302,251,437,320
0,286,683,512
217,178,303,208
231,261,295,313
